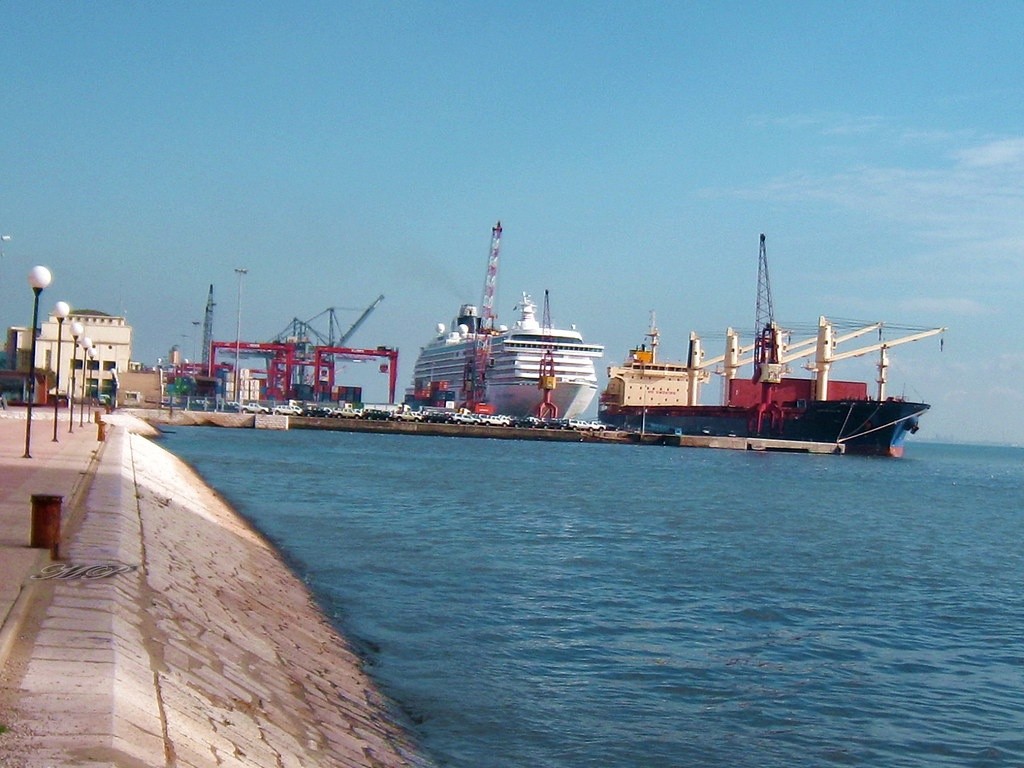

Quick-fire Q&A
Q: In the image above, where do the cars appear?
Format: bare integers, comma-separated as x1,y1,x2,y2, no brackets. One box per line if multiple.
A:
235,399,617,431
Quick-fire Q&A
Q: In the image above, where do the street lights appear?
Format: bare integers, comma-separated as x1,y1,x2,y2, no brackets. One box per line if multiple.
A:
68,322,83,433
86,347,95,422
234,268,248,404
79,337,93,428
53,302,70,445
22,265,52,459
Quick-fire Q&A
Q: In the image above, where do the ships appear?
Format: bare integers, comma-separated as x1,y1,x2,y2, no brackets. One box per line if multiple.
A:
413,293,605,420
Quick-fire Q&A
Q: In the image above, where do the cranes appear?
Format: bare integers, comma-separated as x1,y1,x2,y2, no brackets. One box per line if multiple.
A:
753,233,786,435
201,282,217,377
533,288,559,420
456,220,503,422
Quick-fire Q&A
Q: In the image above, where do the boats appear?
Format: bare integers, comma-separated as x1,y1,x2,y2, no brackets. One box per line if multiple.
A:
598,314,947,460
